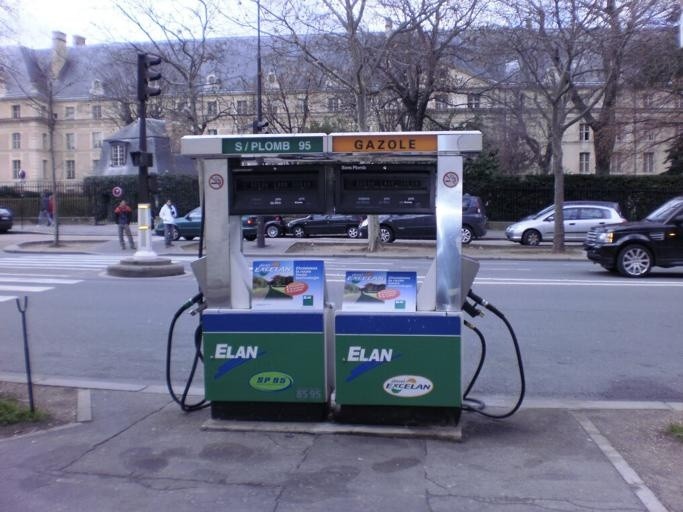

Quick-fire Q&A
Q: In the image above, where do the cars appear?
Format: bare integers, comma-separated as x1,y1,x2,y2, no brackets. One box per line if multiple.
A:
155,206,256,241
518,200,621,233
0,209,13,234
503,205,627,246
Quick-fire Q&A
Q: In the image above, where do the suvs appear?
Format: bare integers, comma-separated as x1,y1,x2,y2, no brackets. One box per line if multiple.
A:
583,196,683,277
357,196,487,244
285,214,366,239
262,216,285,237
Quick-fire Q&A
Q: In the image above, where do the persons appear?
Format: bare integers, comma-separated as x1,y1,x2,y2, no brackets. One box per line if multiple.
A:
46,194,57,225
158,200,178,247
114,199,137,251
36,191,56,227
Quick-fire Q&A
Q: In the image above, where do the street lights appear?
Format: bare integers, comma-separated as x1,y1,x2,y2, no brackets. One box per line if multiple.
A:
18,169,26,231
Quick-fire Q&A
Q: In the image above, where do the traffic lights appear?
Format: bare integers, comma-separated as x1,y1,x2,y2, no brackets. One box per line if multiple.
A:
137,52,162,100
255,120,270,134
